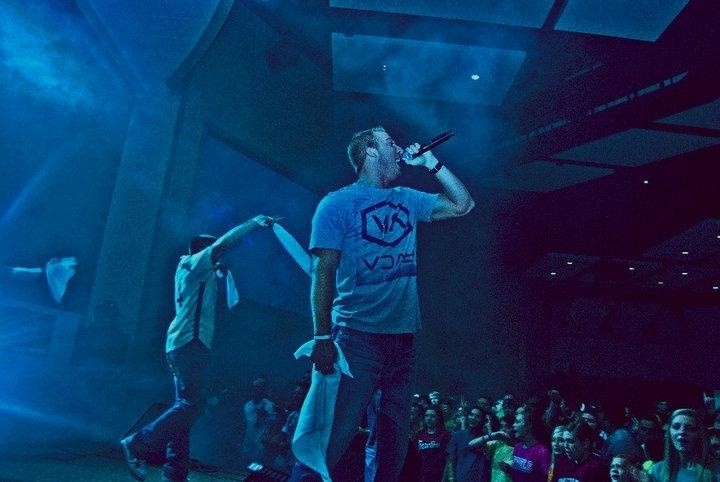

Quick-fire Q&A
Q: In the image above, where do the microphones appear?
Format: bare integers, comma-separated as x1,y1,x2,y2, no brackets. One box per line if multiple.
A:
402,130,454,161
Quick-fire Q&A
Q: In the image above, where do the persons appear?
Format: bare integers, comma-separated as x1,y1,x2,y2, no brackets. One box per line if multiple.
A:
242,374,720,481
122,212,287,481
289,124,475,481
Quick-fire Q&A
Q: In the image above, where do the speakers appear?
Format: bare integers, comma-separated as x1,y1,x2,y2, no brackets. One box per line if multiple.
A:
121,402,217,467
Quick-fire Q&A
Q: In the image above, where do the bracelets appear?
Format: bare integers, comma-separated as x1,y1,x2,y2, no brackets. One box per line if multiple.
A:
429,160,445,174
312,334,332,340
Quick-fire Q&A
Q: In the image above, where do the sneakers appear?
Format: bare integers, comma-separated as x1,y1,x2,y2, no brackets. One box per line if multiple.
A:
161,461,176,482
120,432,146,481
